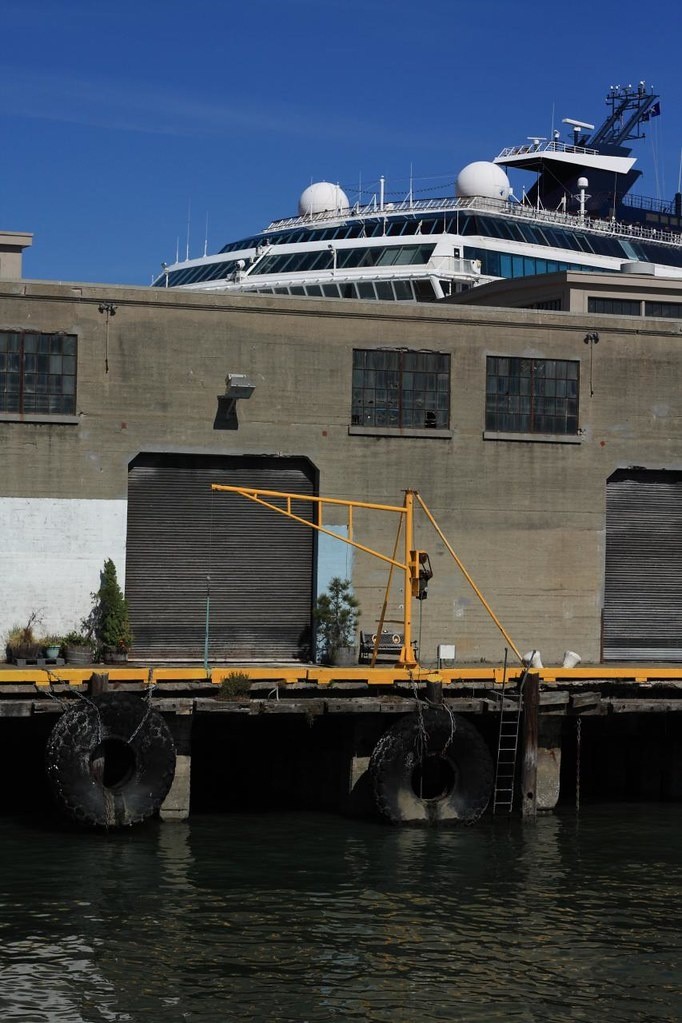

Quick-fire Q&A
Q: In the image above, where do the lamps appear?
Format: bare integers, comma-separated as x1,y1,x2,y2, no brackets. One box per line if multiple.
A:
225,372,255,400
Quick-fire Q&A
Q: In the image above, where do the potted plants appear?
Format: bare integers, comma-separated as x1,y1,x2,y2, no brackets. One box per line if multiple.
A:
43,635,64,659
90,559,133,664
65,628,96,665
7,609,41,659
313,577,361,665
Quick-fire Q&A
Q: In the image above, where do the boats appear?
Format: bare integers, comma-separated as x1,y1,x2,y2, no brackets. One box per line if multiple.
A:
149,82,680,305
